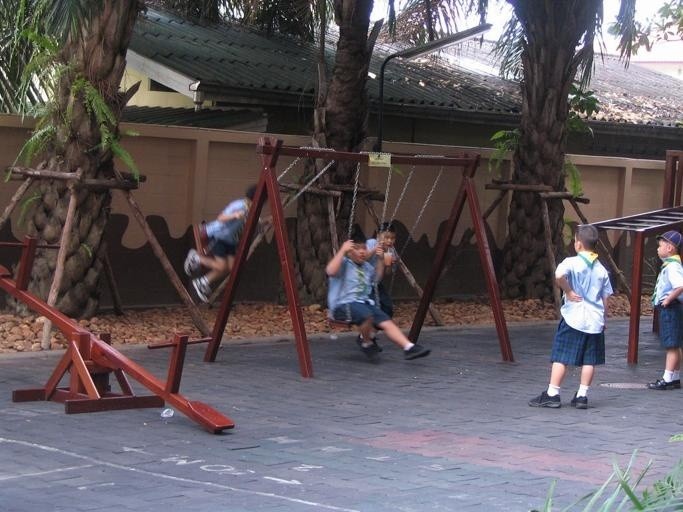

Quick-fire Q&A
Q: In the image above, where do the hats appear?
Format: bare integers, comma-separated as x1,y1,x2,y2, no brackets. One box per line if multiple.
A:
655,230,683,250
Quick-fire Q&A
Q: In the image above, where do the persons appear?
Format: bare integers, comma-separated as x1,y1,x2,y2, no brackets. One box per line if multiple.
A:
324,230,431,361
526,221,613,412
182,178,271,308
355,222,401,354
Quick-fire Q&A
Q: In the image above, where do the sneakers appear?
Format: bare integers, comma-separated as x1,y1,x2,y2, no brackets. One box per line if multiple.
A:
403,344,432,360
528,391,561,408
184,249,200,277
192,276,211,304
570,391,588,409
648,378,681,390
356,334,383,353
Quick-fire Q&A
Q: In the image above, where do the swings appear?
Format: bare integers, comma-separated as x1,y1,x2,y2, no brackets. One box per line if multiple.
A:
325,160,393,330
193,157,335,274
368,164,444,305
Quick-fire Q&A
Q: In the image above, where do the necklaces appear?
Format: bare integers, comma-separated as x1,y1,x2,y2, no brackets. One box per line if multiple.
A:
646,229,681,391
